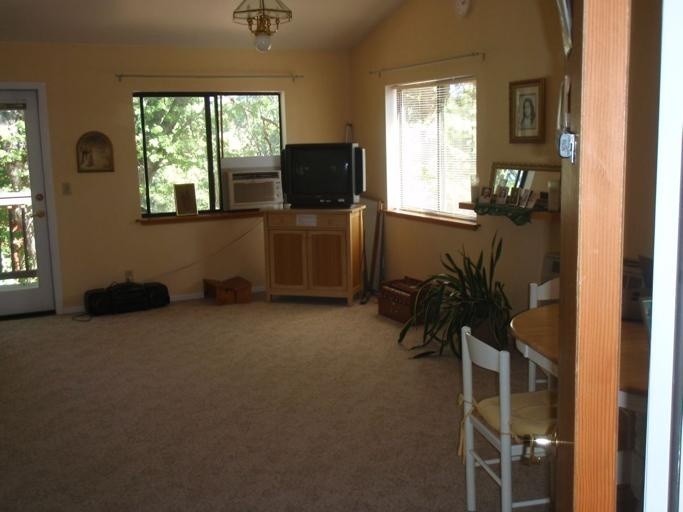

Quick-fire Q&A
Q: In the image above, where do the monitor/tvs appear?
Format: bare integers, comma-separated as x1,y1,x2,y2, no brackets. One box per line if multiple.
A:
279,141,367,210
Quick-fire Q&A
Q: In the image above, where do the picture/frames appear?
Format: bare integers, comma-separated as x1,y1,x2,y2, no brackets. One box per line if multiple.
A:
477,185,540,210
174,183,199,217
73,130,117,176
508,76,547,144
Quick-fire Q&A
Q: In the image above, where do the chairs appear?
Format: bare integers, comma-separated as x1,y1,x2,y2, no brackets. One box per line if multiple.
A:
460,327,635,512
529,277,562,392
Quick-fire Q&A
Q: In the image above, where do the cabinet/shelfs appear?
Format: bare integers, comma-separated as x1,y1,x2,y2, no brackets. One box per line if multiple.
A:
260,203,368,306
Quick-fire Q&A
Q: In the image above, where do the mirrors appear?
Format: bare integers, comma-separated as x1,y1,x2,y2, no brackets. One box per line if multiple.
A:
488,161,561,207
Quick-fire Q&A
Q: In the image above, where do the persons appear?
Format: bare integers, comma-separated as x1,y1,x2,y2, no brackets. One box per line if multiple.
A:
521,99,535,128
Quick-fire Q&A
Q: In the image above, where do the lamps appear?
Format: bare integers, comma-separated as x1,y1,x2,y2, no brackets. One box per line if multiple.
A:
231,0,294,52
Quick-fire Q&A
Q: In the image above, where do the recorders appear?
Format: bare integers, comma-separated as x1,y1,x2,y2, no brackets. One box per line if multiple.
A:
83,280,170,316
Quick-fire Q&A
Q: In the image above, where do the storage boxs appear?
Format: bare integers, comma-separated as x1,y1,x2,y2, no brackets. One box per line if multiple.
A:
376,275,437,327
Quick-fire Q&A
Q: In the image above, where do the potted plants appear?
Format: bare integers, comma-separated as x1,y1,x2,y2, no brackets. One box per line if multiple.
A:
396,230,514,360
